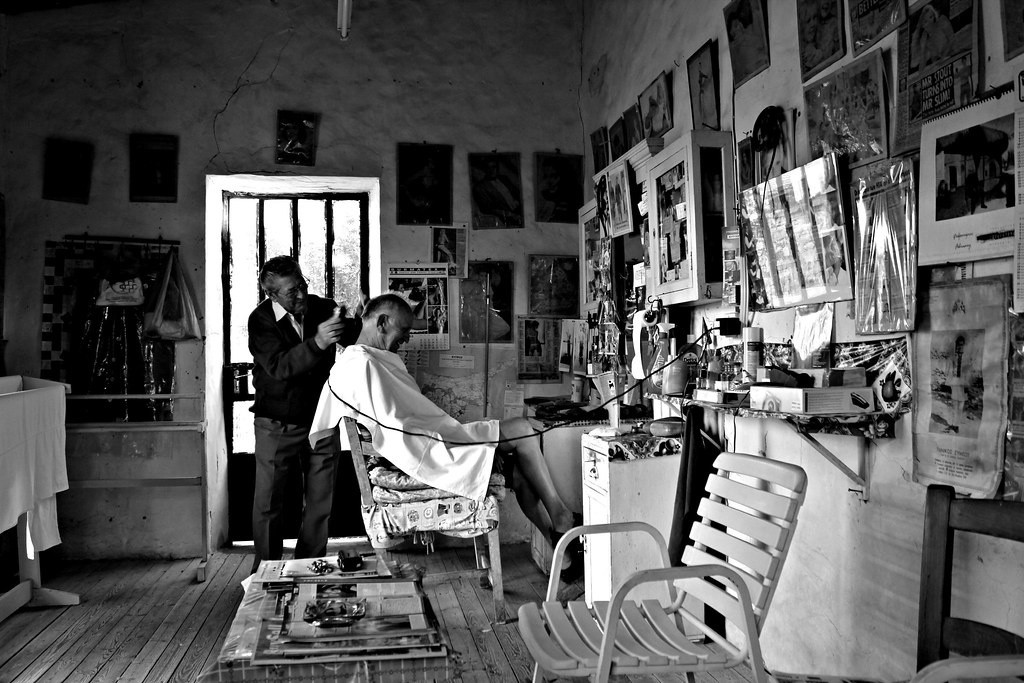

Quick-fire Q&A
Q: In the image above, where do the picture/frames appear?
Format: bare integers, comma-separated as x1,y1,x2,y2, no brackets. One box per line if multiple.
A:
685,37,721,131
396,141,453,226
467,151,525,230
528,254,580,318
638,70,674,137
722,0,770,89
603,159,634,238
794,0,908,172
533,152,584,224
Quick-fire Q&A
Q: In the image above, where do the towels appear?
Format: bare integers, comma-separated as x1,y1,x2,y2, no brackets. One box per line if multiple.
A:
0,385,69,561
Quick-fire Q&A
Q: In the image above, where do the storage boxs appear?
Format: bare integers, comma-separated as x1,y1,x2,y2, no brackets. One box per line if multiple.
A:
750,386,874,415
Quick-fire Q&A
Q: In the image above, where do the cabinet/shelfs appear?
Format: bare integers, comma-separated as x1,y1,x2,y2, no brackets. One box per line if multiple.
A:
581,433,685,608
528,419,620,575
578,130,736,322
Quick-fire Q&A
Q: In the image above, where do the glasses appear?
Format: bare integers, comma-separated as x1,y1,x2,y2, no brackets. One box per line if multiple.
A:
269,274,311,299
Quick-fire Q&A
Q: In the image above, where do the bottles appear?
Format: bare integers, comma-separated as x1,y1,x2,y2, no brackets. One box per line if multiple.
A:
662,356,687,395
571,377,583,403
743,327,759,381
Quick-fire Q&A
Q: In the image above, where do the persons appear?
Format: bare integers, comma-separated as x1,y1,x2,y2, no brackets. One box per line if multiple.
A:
953,56,972,106
437,229,459,271
525,320,543,357
797,0,839,69
910,83,923,120
308,294,577,550
965,160,1015,214
914,6,956,72
249,255,363,575
608,175,627,221
944,335,972,434
725,15,765,52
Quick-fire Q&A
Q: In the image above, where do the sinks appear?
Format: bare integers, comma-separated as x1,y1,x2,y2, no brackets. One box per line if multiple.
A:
63,422,207,478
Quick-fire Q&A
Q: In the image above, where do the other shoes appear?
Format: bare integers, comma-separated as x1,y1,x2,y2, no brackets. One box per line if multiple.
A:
561,561,585,585
550,511,585,551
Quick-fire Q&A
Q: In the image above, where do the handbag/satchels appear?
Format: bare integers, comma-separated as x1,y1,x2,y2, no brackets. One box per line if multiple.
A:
152,254,201,341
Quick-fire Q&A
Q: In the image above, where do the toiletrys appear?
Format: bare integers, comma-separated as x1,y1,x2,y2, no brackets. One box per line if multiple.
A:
570,376,584,402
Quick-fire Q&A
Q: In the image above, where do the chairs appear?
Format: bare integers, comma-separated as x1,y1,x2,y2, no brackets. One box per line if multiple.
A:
342,411,506,625
914,484,1024,683
518,453,807,683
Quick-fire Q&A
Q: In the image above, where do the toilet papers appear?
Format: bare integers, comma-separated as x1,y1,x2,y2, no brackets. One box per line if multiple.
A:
632,310,658,327
